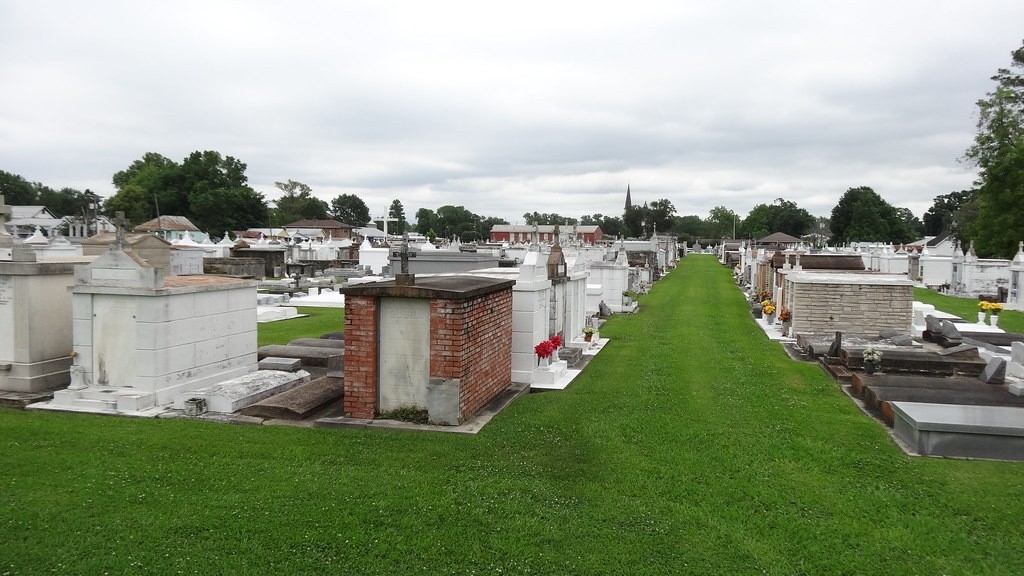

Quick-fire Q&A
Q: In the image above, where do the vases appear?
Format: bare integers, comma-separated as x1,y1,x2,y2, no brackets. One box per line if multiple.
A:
552,350,560,362
766,314,774,326
782,322,792,337
536,356,552,371
987,315,998,328
977,312,986,326
585,336,591,342
863,361,877,375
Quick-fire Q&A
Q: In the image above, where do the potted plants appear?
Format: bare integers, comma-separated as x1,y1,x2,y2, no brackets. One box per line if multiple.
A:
801,216,834,254
751,302,763,319
622,289,637,306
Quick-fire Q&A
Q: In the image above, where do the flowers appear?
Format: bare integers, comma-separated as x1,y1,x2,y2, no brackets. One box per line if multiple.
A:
777,305,793,323
978,301,990,312
989,303,1005,315
581,326,597,337
764,306,776,315
861,345,884,363
534,339,555,366
760,289,773,297
761,300,777,306
549,335,565,351
